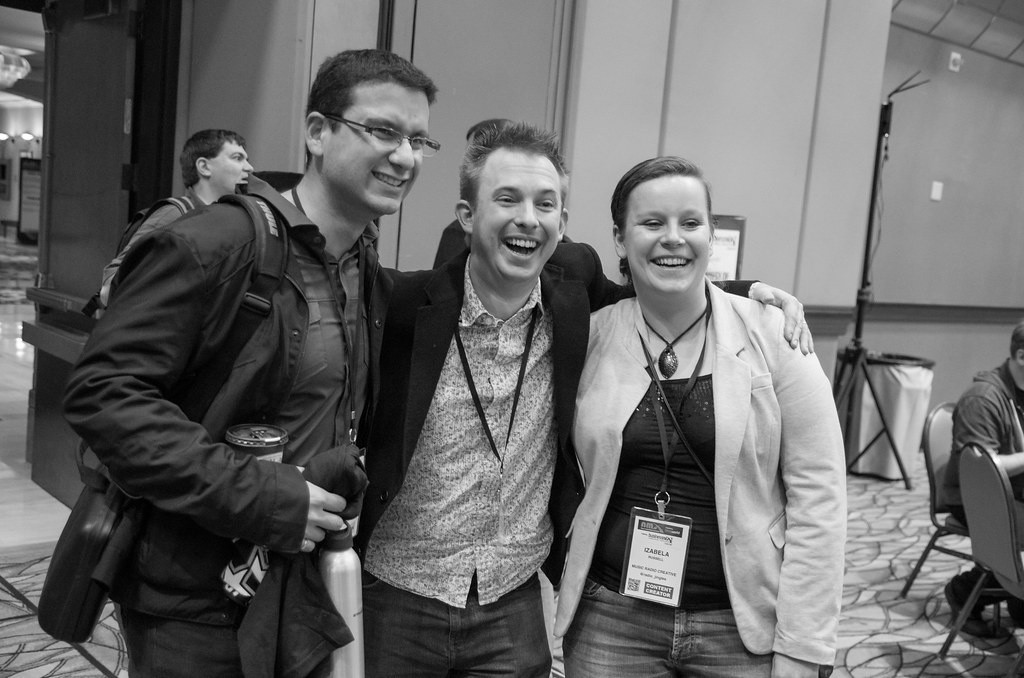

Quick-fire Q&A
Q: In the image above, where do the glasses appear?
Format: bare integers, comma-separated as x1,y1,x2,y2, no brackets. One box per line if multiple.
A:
326,114,440,156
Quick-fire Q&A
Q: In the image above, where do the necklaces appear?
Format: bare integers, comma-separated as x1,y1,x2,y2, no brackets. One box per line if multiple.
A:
637,292,709,379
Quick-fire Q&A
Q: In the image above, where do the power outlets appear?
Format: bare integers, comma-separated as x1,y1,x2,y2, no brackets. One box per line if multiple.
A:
931,181,944,202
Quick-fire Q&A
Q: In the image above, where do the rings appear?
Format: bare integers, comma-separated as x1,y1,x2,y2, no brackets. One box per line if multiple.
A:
802,321,806,324
300,539,308,550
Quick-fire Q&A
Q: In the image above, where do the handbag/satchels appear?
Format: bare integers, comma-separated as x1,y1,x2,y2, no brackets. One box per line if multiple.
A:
38,437,130,642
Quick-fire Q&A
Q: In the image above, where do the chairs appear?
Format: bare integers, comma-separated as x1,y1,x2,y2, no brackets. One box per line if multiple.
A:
900,400,973,599
937,440,1024,677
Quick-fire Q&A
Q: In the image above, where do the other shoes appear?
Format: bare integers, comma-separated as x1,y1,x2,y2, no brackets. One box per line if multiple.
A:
945,575,989,637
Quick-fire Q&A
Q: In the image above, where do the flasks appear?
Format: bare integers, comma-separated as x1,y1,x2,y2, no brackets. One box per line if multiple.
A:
320,519,365,678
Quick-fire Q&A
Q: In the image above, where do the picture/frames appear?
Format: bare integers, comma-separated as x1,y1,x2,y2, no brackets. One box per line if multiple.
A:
705,214,746,282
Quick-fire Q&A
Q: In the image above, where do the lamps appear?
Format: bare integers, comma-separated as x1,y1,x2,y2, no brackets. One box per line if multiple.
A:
0,45,30,87
21,132,40,143
0,132,14,143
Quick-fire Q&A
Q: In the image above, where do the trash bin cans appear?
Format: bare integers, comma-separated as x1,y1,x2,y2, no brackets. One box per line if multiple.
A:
833,348,936,483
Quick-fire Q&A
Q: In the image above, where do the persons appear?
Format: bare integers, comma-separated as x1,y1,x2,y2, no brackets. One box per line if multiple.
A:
58,48,848,678
943,324,1024,639
92,129,254,319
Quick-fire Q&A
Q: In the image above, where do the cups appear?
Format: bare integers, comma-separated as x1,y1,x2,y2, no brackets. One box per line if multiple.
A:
227,423,288,462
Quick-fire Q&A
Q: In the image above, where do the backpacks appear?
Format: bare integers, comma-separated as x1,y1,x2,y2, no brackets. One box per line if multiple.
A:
81,196,194,318
